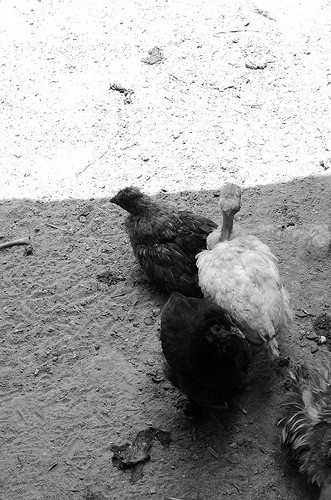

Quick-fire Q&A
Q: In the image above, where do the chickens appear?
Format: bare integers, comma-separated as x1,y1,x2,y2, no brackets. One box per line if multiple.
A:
160,291,248,416
109,187,218,298
194,181,296,363
